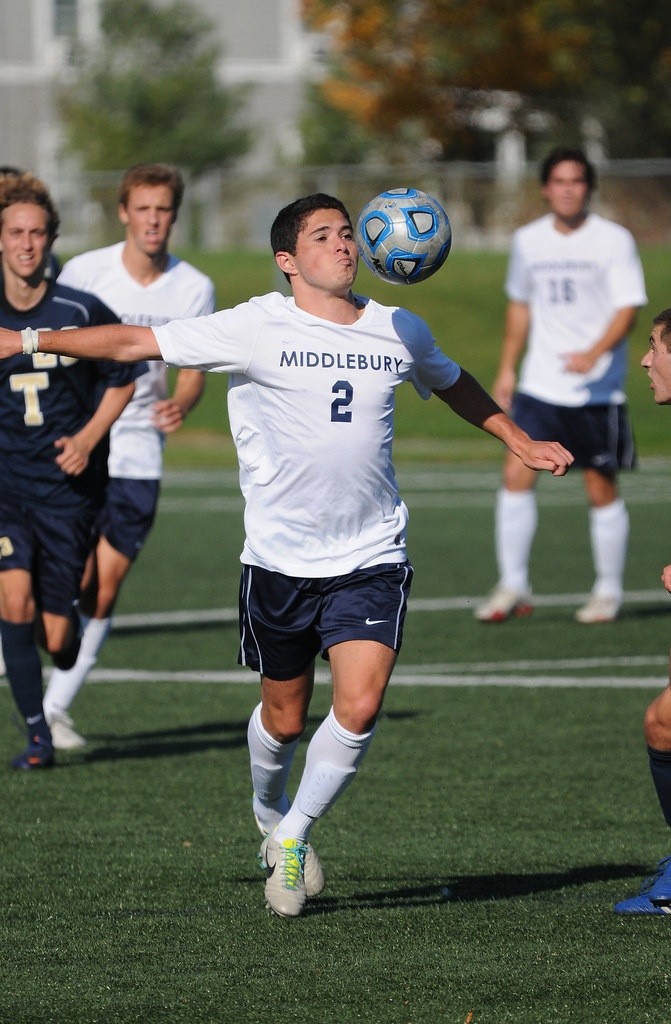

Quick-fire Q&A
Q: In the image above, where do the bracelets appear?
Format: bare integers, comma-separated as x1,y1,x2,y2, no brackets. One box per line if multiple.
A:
21,327,38,354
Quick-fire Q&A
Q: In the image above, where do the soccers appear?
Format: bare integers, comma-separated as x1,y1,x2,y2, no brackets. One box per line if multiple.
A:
354,187,454,285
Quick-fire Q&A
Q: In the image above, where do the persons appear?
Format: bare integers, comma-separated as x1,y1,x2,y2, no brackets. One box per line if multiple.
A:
0,192,577,918
42,161,215,747
612,309,671,914
472,145,649,626
0,172,150,770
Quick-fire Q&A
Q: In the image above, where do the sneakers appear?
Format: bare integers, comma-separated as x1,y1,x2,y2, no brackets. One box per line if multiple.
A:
9,715,57,771
613,871,671,916
574,590,624,624
255,825,312,919
471,582,538,623
46,619,86,672
46,706,86,752
253,794,326,898
650,856,671,906
